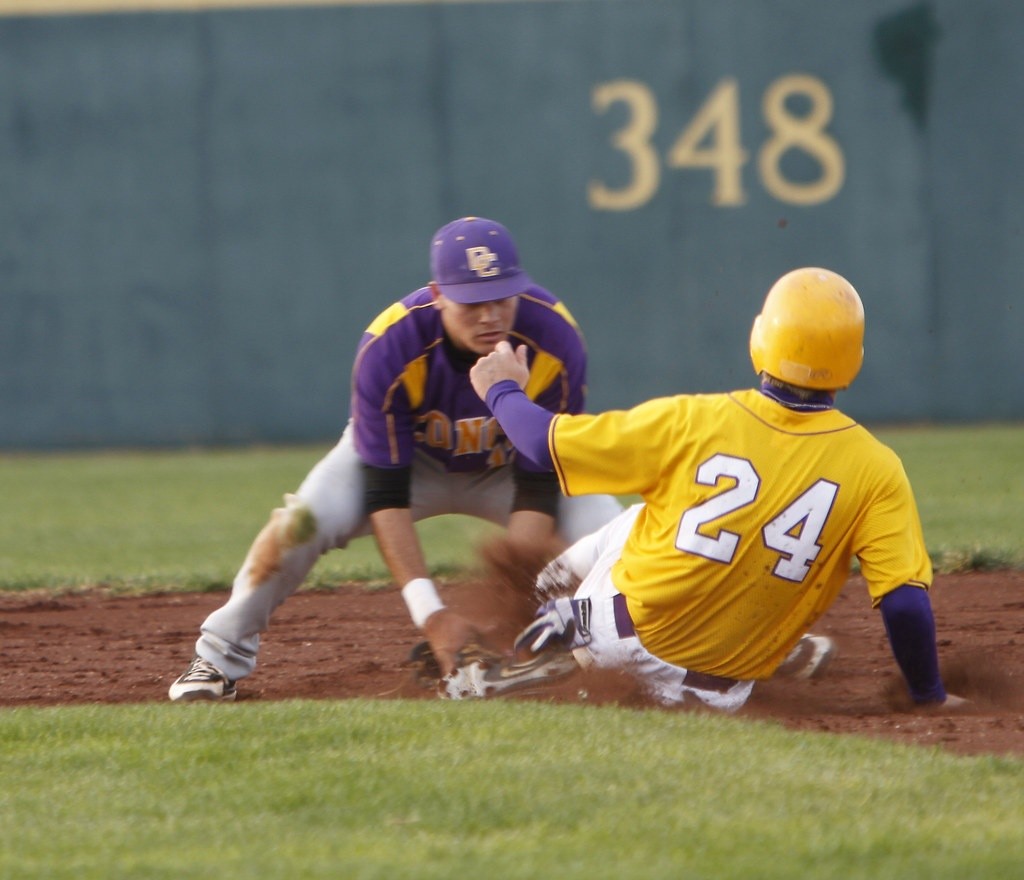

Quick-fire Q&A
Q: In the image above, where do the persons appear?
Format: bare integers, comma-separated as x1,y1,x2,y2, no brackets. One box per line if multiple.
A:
168,216,627,703
469,267,968,714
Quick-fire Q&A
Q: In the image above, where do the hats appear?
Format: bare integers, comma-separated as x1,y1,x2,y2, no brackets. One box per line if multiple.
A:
430,216,531,303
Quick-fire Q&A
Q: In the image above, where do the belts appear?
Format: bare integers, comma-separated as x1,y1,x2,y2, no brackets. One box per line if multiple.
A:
612,592,737,694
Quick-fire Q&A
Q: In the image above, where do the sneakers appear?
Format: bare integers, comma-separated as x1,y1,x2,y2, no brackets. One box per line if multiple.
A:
169,655,238,702
437,650,584,700
773,632,833,684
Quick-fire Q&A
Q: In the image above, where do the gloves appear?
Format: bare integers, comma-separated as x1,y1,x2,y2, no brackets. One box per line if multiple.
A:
513,599,593,651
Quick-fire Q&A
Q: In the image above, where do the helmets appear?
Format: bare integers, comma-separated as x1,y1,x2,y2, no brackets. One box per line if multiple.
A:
749,266,864,389
